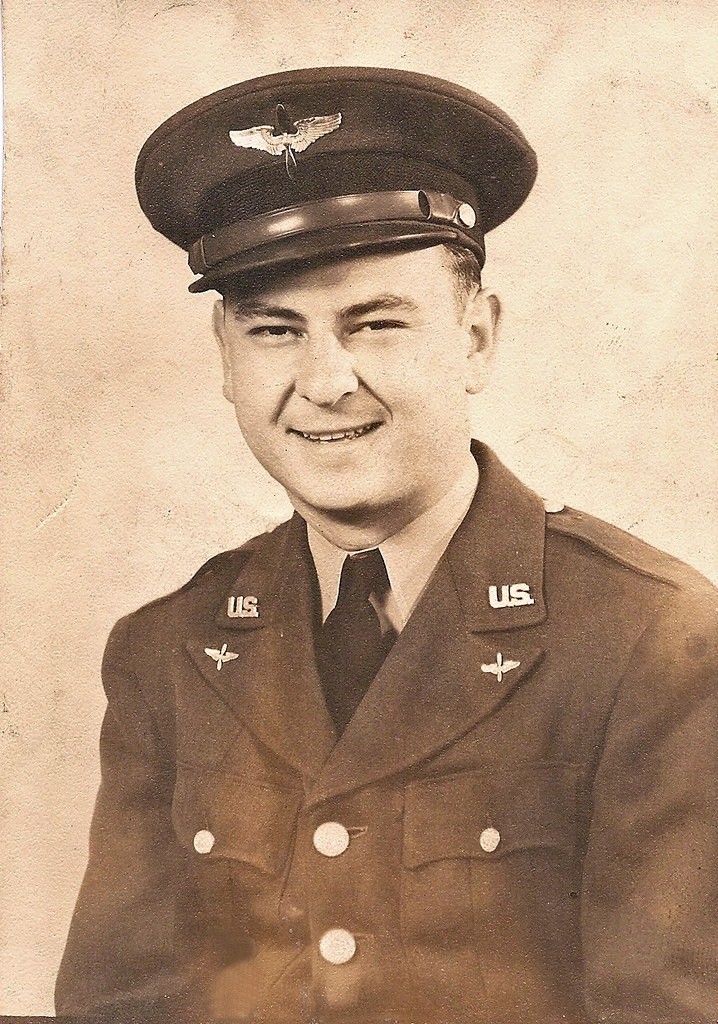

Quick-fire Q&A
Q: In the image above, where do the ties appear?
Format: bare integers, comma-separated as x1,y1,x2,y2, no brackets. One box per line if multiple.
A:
317,549,386,727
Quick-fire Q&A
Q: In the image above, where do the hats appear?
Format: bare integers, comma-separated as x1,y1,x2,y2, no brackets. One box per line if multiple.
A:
135,65,539,293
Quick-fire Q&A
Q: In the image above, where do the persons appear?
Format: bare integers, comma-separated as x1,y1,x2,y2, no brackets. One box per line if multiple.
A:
50,65,718,1024
